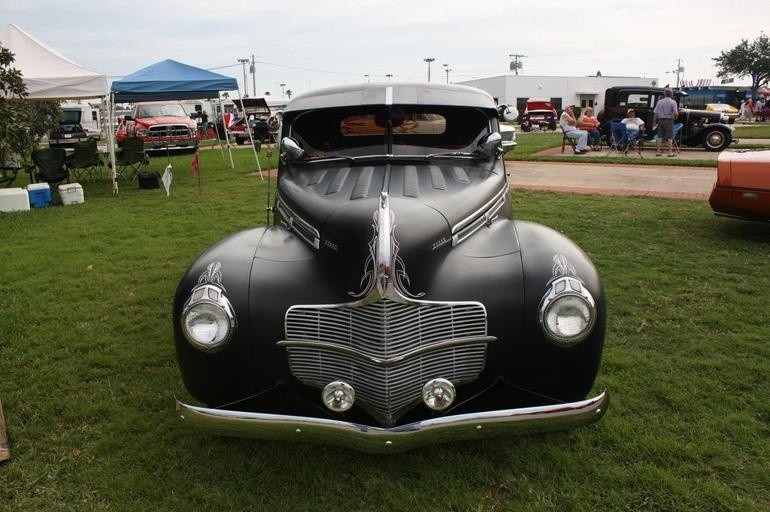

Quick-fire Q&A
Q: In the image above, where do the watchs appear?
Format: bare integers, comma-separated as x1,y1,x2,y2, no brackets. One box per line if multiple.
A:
198,109,211,138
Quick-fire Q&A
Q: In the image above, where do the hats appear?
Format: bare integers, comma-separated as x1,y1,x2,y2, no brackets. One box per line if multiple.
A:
626,109,635,114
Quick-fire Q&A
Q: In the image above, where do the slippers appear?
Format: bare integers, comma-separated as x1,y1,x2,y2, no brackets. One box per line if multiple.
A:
655,153,677,157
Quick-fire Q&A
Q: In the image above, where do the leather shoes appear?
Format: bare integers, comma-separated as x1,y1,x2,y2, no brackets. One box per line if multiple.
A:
575,148,590,155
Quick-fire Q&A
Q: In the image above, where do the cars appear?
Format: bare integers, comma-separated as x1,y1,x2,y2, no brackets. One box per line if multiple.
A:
171,81,610,455
700,104,739,125
521,97,557,131
49,101,199,155
709,148,770,223
227,97,284,145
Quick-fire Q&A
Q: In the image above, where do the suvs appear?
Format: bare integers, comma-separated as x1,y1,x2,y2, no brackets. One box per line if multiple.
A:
596,85,732,152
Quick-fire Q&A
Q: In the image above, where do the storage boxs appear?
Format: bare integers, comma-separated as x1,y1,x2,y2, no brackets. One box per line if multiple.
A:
138,170,160,189
0,182,85,212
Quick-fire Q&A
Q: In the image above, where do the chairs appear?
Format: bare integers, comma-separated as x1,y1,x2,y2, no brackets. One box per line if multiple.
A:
559,118,684,158
0,136,151,195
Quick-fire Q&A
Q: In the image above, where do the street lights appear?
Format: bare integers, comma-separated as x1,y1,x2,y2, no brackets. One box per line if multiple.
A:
237,58,250,95
424,58,435,83
280,84,287,99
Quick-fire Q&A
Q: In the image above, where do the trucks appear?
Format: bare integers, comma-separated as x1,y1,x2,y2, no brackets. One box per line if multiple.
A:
211,99,235,125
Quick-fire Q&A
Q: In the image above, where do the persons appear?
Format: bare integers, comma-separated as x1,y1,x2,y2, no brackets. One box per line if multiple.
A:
559,104,590,154
577,106,603,151
651,87,679,158
736,96,768,122
620,108,646,146
